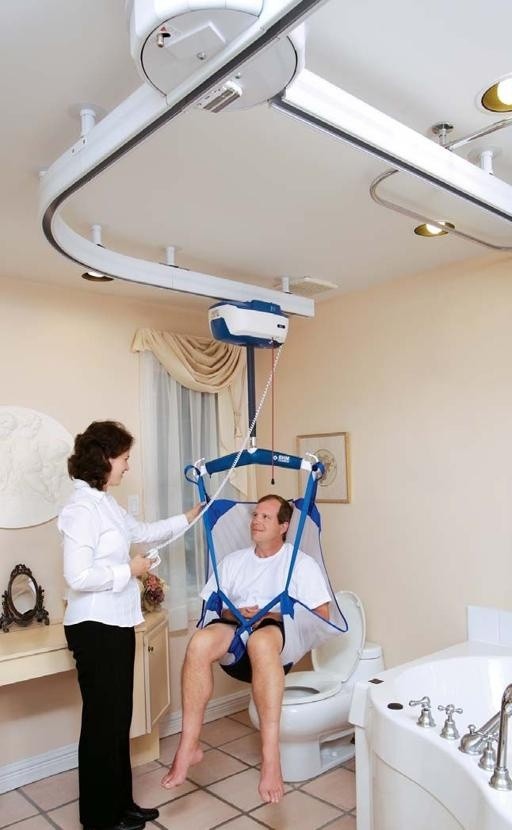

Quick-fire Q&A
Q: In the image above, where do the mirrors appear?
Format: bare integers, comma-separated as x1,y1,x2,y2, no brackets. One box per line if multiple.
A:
0,564,49,633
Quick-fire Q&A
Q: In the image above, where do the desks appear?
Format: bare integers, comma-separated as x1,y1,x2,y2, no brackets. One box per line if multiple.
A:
0,608,172,795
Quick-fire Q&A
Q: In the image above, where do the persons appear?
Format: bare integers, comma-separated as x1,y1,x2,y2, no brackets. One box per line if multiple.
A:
159,491,334,803
56,418,208,830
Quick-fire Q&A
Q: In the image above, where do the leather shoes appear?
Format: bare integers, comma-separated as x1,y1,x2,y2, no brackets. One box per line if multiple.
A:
125,802,159,821
83,815,146,830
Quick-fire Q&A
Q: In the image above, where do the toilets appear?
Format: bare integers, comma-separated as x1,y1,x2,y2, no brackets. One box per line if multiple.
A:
249,588,386,781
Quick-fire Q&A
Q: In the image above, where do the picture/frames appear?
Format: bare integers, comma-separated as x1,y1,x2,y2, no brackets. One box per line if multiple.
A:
296,432,351,503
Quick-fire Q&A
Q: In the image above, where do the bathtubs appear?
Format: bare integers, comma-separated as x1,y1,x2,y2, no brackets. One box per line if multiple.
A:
348,602,512,828
